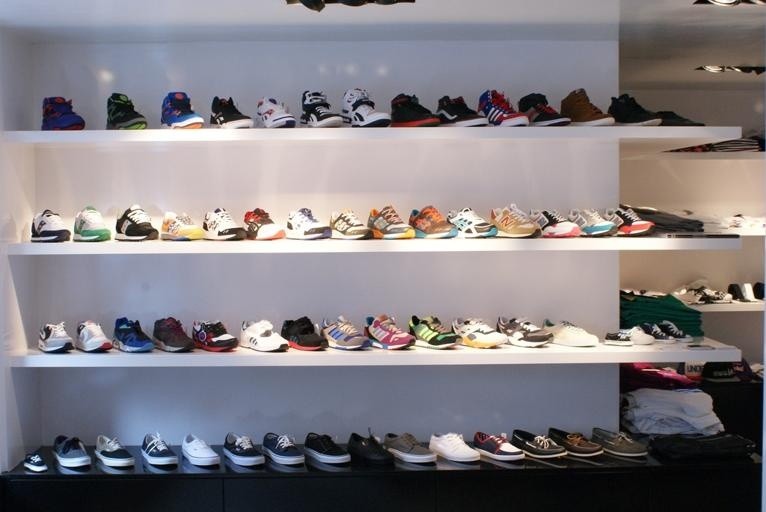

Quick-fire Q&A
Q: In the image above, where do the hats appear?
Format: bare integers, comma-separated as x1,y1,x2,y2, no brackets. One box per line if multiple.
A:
702,357,763,383
677,361,707,381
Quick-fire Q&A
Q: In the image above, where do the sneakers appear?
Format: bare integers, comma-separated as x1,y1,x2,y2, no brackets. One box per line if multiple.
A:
72,206,112,242
160,91,205,128
341,87,392,126
160,203,656,241
208,94,253,129
391,88,663,126
21,452,48,473
51,433,91,470
40,96,86,130
603,318,695,346
299,90,344,129
106,92,148,129
140,430,179,469
221,426,649,468
254,96,297,128
93,434,136,470
29,209,70,243
114,203,158,240
237,313,601,354
181,430,220,467
35,315,239,353
